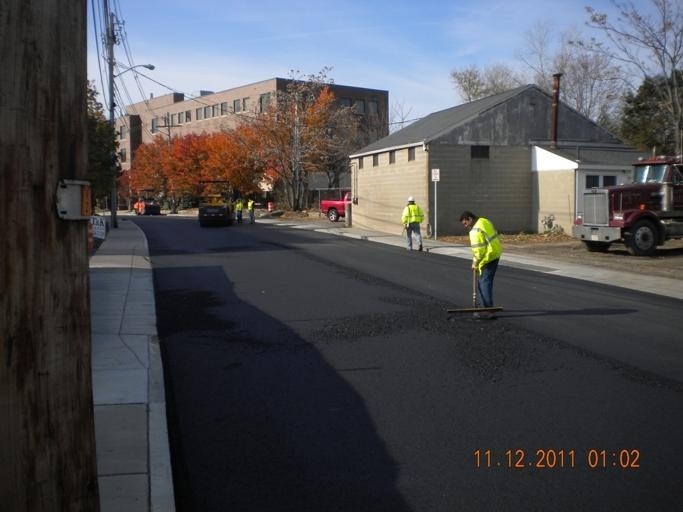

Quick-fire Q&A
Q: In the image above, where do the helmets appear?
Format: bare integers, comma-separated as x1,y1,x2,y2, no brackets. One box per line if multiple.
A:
408,197,414,201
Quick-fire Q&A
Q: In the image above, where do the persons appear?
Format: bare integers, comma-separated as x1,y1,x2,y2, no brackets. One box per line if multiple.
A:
247,196,254,224
400,195,424,252
457,209,502,320
234,198,243,225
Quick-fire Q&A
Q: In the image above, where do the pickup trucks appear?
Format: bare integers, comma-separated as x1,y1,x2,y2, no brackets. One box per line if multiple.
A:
321,192,351,222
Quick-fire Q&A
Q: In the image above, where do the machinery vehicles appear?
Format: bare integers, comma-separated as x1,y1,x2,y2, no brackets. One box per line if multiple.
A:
134,188,160,216
198,193,235,227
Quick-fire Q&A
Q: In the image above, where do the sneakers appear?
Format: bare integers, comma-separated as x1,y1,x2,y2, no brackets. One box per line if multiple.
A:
419,244,422,251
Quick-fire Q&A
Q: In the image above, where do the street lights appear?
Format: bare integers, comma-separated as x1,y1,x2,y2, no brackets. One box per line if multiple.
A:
107,63,154,226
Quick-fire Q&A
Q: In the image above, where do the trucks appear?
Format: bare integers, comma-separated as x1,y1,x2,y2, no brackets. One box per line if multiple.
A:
573,155,683,256
